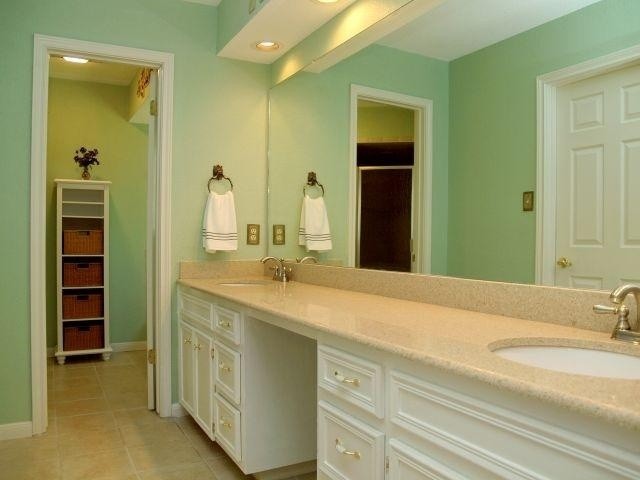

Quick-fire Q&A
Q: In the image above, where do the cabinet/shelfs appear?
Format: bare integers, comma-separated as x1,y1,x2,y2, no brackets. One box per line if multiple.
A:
52,178,114,366
384,329,639,479
213,294,318,476
175,281,214,442
318,328,385,480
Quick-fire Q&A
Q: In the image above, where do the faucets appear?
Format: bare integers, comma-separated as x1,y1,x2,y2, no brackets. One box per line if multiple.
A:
610,284,640,326
300,256,317,264
260,256,283,277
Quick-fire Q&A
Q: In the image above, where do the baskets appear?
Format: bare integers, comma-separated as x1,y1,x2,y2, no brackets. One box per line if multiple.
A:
63,294,103,319
63,229,103,255
63,325,104,351
63,262,103,287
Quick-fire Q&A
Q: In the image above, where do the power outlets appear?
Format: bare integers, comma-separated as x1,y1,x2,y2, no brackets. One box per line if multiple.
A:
272,224,287,246
246,224,262,246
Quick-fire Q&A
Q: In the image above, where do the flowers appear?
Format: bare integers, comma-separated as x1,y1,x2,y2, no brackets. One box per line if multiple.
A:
74,146,100,170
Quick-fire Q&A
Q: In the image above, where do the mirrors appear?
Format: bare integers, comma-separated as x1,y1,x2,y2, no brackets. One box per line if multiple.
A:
264,0,640,295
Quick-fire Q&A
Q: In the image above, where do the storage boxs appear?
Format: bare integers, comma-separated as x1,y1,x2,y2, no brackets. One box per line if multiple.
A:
62,230,105,352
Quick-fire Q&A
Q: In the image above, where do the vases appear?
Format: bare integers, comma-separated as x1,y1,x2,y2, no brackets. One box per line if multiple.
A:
81,167,92,180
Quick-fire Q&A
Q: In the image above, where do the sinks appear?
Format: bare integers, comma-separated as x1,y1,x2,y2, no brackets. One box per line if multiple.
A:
487,337,640,380
217,280,270,287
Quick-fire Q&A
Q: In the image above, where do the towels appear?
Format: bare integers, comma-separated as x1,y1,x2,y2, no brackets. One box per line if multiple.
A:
297,194,334,252
201,190,241,254
